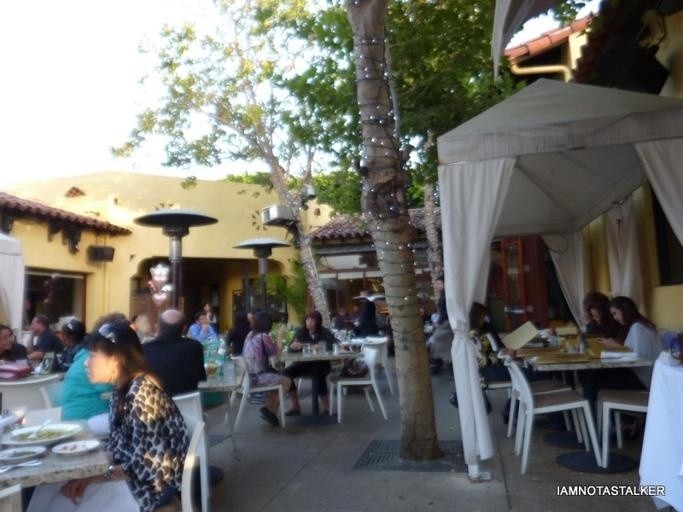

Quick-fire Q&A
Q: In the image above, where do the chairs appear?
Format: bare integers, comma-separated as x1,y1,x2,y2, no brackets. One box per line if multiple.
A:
478,323,682,512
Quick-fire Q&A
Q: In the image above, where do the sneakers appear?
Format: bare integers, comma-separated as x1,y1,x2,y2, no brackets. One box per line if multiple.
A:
260,407,278,426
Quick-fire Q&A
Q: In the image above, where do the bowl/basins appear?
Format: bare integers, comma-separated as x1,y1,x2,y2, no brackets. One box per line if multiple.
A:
2,422,82,449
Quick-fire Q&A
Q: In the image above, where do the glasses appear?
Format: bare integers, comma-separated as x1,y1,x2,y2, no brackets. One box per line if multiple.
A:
98,325,118,344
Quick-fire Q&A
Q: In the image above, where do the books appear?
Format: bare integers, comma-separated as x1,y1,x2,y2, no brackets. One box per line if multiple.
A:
584,337,612,353
500,319,539,352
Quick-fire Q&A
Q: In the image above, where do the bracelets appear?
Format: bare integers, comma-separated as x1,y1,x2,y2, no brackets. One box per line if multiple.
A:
103,466,114,481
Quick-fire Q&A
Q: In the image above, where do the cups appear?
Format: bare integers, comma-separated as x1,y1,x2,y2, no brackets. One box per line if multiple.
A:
302,344,310,360
207,364,217,385
222,361,237,385
671,332,683,366
313,345,320,355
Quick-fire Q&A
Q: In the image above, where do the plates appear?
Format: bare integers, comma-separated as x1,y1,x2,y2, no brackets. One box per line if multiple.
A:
51,440,100,457
1,446,47,463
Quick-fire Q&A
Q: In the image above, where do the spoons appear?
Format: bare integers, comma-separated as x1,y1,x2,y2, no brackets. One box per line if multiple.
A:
28,419,52,439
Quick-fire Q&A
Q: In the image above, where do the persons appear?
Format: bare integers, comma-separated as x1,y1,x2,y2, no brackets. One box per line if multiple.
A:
139,309,207,396
0,323,26,363
59,311,121,423
186,311,217,344
334,305,348,330
55,318,87,370
23,322,190,512
433,275,448,325
201,302,217,335
128,315,140,333
582,295,663,443
347,304,361,320
240,312,292,426
281,311,341,417
25,314,63,365
419,307,427,323
225,311,255,355
582,291,626,343
467,301,536,425
109,312,139,329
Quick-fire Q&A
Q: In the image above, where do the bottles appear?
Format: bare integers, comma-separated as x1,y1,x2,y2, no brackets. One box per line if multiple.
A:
550,324,557,348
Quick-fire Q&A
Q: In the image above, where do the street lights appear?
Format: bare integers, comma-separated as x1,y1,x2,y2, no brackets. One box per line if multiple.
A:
134,207,217,312
231,237,290,319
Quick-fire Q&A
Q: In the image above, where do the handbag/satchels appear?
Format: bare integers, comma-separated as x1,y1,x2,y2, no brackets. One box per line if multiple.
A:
343,358,367,378
265,365,279,374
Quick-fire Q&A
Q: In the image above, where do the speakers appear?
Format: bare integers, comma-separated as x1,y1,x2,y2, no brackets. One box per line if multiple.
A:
88,244,115,263
